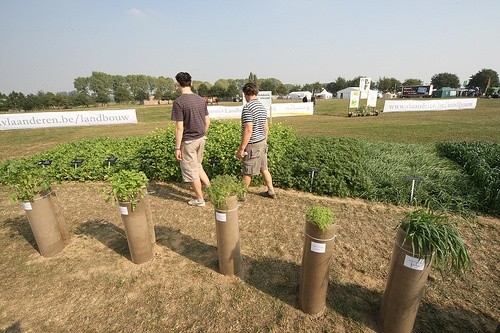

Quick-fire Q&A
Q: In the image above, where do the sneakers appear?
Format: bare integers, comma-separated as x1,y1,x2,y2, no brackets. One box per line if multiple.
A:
188,199,205,206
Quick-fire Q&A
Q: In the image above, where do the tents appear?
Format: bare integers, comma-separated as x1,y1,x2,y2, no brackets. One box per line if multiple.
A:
314,88,333,99
289,91,312,100
433,86,480,98
384,92,397,99
336,87,371,99
372,88,383,99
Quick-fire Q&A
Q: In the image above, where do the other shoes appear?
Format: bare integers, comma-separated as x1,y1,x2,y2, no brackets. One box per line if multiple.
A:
260,190,277,199
237,196,247,203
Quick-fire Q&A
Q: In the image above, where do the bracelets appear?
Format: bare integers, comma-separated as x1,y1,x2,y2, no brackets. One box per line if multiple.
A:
174,148,180,150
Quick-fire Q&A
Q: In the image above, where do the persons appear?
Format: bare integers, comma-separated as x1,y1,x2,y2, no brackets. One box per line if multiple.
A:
170,72,211,207
234,83,277,200
482,91,500,100
302,94,308,102
203,97,209,105
310,94,316,107
215,97,219,105
